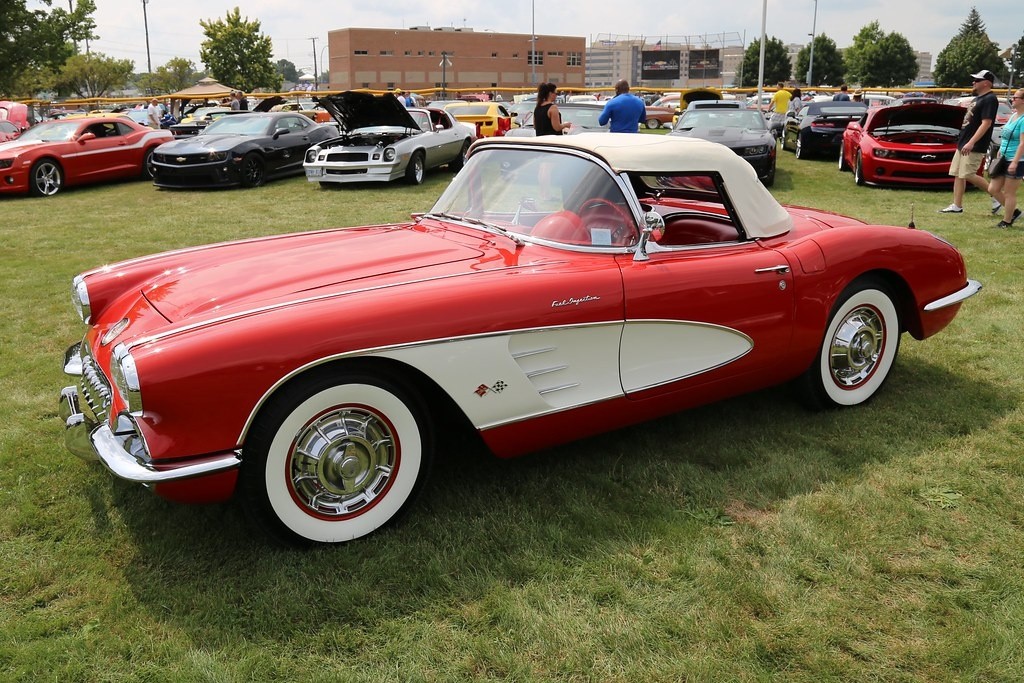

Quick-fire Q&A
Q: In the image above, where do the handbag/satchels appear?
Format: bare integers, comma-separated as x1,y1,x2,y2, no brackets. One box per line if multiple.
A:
988,158,1004,177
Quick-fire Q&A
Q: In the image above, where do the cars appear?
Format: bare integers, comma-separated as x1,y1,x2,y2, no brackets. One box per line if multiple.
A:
442,102,521,140
0,82,1024,129
780,100,870,160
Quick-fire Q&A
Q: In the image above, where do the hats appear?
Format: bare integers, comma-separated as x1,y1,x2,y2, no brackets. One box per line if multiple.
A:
970,69,994,82
852,88,863,96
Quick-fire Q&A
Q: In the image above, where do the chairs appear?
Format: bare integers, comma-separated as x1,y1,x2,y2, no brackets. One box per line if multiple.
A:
658,212,739,245
576,203,655,245
278,119,289,128
89,124,105,137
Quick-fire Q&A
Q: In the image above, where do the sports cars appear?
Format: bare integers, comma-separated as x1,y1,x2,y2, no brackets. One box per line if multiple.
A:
151,111,340,189
302,88,477,188
58,128,984,551
503,102,611,138
664,108,782,187
0,117,176,199
838,103,986,190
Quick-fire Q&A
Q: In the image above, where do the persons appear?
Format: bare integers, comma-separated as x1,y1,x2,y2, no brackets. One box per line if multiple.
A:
237,92,248,110
219,91,240,110
455,91,461,98
488,90,494,100
147,99,162,129
533,83,572,201
788,89,804,113
832,85,850,101
77,105,85,112
598,79,647,133
988,87,1024,229
768,82,792,127
395,88,416,107
850,89,863,103
937,70,1003,214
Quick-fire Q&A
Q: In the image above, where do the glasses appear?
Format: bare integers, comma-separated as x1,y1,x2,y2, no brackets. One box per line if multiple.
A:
973,78,987,82
550,91,559,95
1011,96,1024,101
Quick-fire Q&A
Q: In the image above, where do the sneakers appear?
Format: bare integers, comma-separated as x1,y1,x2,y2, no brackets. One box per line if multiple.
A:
997,220,1011,228
990,195,1002,213
938,203,963,213
1012,209,1022,222
